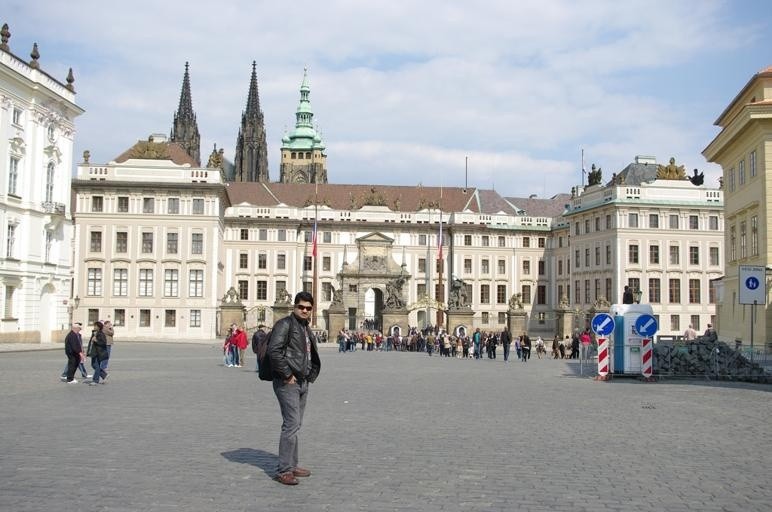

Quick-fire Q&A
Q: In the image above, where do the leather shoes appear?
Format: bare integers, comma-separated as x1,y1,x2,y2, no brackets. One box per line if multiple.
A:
277,467,297,485
292,467,311,477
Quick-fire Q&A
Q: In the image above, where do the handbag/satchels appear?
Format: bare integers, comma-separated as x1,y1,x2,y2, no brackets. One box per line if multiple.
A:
94,345,109,361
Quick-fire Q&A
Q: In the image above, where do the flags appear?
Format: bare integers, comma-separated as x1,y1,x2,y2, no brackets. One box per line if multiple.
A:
313,204,317,256
437,212,442,260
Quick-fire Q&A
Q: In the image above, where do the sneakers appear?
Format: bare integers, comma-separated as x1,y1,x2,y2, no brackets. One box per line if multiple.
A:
61,374,109,385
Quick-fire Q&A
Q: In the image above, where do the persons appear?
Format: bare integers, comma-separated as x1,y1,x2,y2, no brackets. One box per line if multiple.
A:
60,320,114,385
336,319,591,362
315,331,327,343
268,292,320,484
224,322,267,371
705,324,718,343
683,324,696,340
623,285,633,304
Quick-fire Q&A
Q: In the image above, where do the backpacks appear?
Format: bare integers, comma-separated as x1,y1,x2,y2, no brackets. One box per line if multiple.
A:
256,317,293,379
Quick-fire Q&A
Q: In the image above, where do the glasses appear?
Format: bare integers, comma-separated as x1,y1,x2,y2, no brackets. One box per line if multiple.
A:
298,306,312,311
